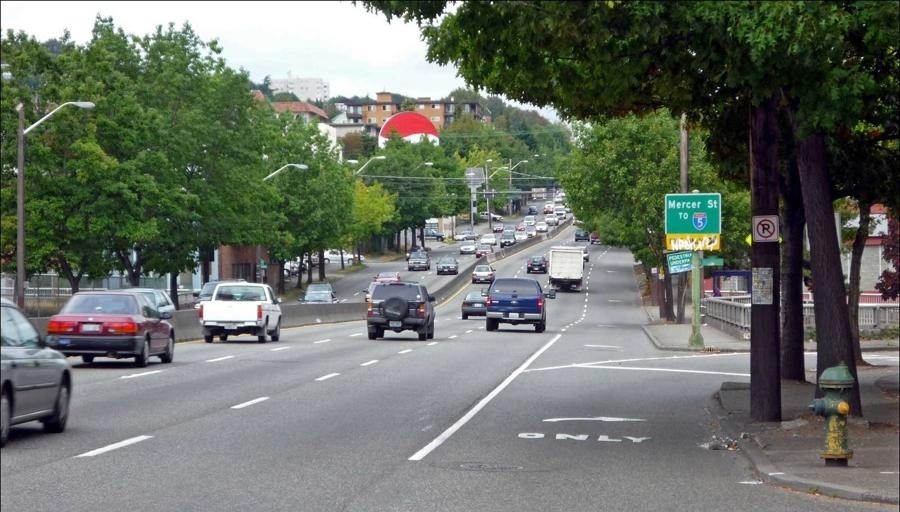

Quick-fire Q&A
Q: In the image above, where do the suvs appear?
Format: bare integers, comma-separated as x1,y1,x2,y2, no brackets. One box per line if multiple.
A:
575,228,589,242
192,278,248,310
481,277,556,333
365,280,436,341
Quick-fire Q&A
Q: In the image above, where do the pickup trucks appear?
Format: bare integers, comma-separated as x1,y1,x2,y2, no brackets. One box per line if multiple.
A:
200,282,282,342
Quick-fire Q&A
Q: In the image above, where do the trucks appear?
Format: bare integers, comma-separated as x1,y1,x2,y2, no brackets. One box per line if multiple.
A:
548,245,585,292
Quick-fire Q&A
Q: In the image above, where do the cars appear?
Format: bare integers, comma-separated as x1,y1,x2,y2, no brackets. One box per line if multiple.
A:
1,297,72,448
323,247,365,264
461,289,488,319
405,188,571,283
297,290,339,304
46,289,174,367
574,245,589,263
104,287,176,313
590,230,604,244
363,282,383,302
300,281,336,295
372,271,401,283
283,252,320,278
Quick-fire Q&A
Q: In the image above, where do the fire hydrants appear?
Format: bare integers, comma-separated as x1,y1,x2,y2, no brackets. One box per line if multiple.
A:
809,360,854,467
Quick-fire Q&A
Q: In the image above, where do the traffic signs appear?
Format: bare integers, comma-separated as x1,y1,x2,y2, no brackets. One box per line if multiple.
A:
665,193,722,236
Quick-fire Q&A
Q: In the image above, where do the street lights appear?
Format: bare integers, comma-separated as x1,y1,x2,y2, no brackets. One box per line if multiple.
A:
17,101,95,310
318,159,359,178
255,164,308,283
350,156,386,176
403,162,433,174
467,153,547,224
